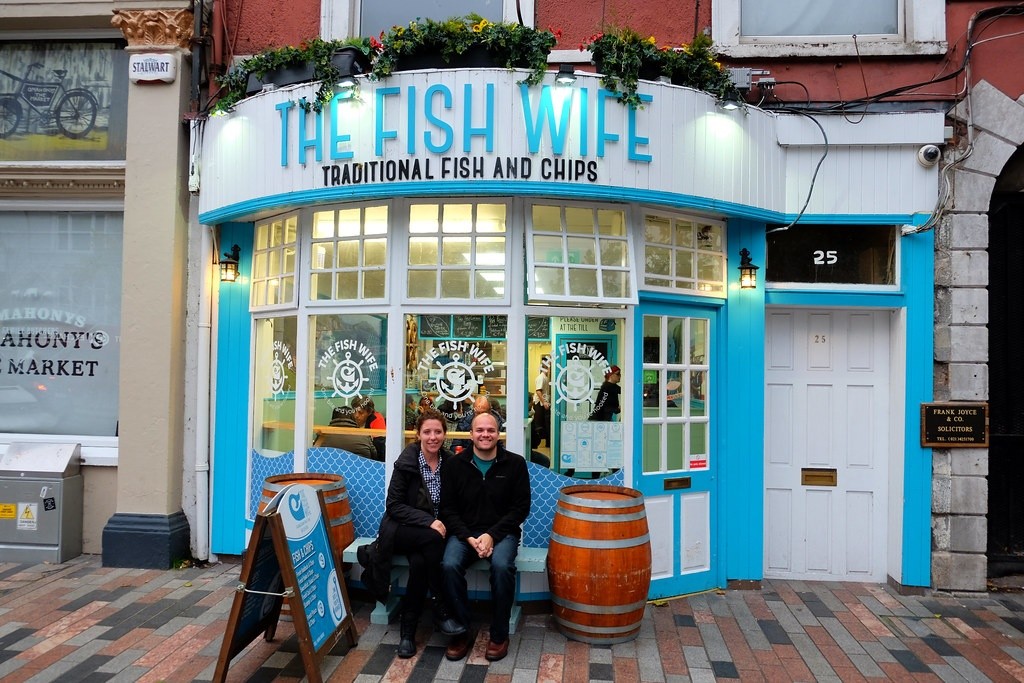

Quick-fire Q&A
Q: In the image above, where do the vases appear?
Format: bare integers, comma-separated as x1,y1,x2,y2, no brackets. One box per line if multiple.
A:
400,45,512,71
245,47,368,97
592,51,707,89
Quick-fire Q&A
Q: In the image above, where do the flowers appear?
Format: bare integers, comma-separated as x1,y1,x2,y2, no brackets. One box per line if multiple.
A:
208,12,745,121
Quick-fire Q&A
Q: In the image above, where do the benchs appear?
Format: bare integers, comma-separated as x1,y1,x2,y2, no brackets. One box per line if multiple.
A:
343,537,549,637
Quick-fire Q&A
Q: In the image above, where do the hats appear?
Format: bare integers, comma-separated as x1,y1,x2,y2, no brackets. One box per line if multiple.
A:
406,395,413,405
604,365,619,380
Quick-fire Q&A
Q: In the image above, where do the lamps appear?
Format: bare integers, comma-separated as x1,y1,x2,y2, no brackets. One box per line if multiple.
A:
218,244,242,282
737,248,759,289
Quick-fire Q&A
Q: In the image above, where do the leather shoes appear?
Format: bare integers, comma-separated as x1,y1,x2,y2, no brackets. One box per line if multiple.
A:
485,632,509,661
446,633,473,661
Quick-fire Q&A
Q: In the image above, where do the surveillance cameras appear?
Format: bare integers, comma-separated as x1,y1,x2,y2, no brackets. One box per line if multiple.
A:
918,145,942,167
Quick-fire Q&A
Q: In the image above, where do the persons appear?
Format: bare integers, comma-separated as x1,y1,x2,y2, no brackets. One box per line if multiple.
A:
441,412,531,660
564,365,621,479
380,410,454,658
313,357,551,460
643,382,677,408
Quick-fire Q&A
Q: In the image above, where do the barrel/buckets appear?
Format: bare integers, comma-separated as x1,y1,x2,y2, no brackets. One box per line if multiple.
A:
547,484,653,643
257,473,355,622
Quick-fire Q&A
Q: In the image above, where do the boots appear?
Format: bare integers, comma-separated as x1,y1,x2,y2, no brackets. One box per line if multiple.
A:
397,608,417,657
431,595,466,635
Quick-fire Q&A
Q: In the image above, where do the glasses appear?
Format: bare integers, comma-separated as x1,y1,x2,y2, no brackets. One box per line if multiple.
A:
614,371,621,377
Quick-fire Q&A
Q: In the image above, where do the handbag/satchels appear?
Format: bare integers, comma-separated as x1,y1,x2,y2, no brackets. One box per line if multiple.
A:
357,537,383,568
